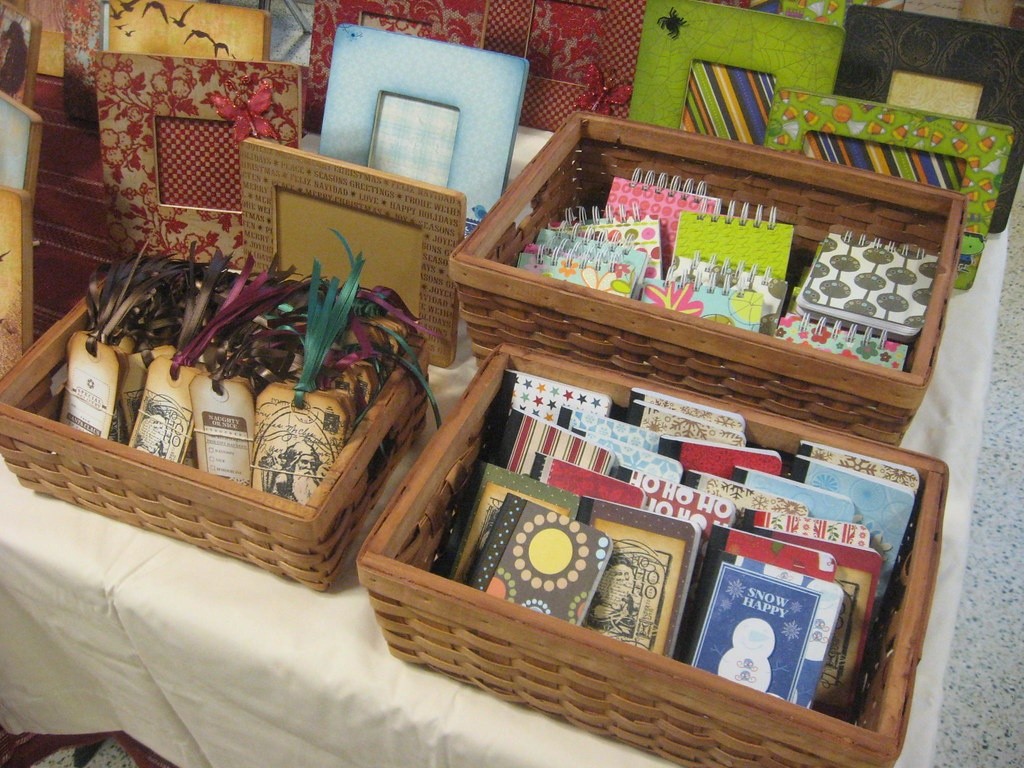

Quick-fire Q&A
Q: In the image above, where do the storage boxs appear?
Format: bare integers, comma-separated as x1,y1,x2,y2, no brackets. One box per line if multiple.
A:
0,261,434,595
445,110,968,451
355,339,950,768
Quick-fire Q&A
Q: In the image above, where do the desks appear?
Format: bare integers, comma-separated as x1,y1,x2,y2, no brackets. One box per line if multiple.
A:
0,209,1013,768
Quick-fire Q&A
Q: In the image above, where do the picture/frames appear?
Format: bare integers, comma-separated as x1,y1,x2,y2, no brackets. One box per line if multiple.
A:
301,0,490,138
237,135,468,370
759,86,1014,288
872,1,1022,32
11,0,65,77
99,0,272,63
0,0,43,111
626,0,846,155
318,22,530,242
0,186,35,378
483,1,647,135
0,90,43,213
91,51,306,272
832,6,1023,235
703,0,868,29
61,1,110,131
